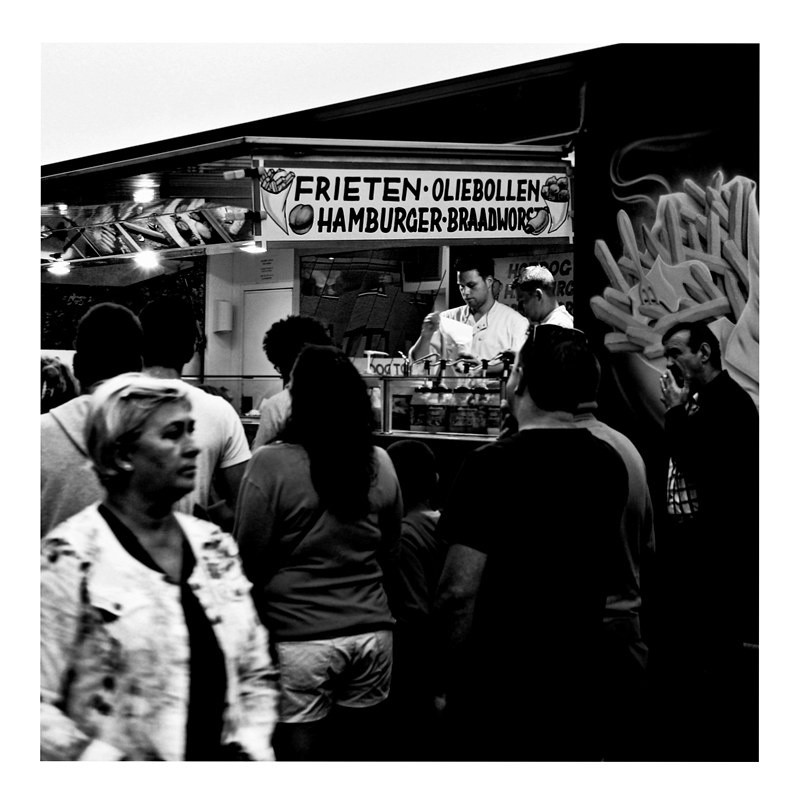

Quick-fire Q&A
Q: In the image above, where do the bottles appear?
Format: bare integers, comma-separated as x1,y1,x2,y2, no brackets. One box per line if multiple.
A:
410,386,501,434
367,384,382,432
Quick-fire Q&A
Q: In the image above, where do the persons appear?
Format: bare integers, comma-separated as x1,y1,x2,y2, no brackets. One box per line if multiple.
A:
40,296,252,530
512,263,577,336
384,440,458,715
439,324,660,761
40,384,279,762
659,322,759,761
254,314,335,451
234,345,407,761
411,253,529,376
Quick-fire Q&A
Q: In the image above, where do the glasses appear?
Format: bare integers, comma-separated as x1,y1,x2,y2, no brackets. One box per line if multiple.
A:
532,324,588,352
